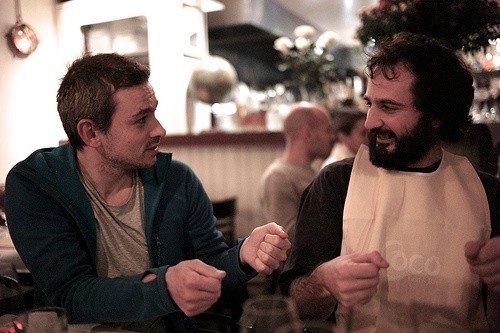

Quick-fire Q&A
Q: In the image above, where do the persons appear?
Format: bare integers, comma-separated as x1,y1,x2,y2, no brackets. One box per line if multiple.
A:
278,33,500,333
4,54,290,333
256,103,337,292
321,109,369,167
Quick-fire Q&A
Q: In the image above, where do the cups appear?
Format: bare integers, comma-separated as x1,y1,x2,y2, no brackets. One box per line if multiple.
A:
12,307,68,333
239,296,299,333
